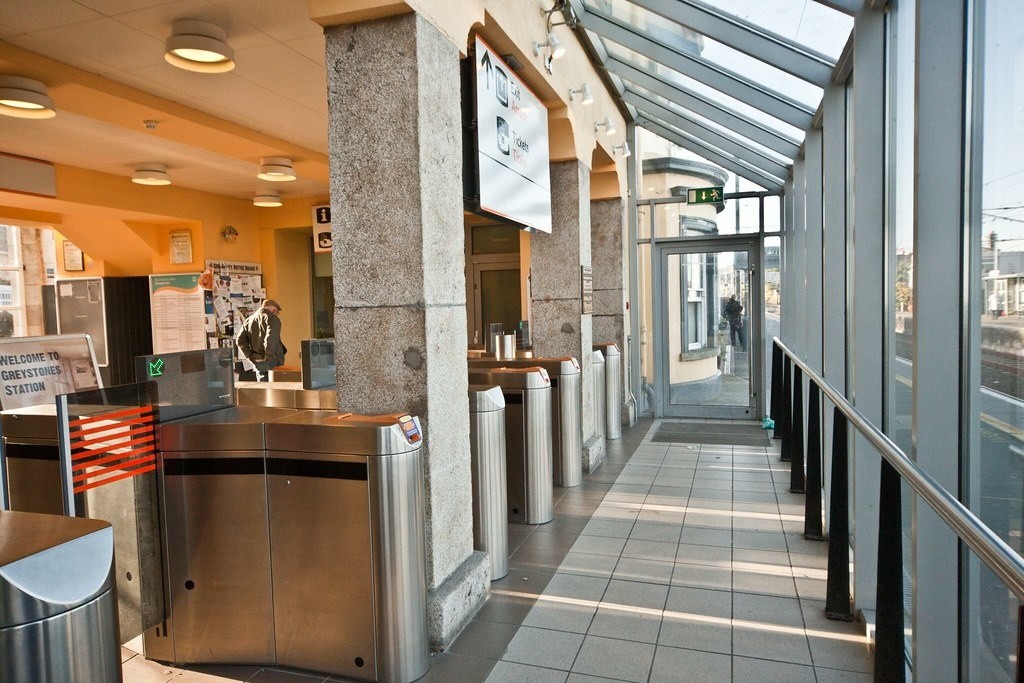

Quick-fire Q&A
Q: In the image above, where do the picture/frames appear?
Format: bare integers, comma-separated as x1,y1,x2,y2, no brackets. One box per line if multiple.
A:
63,239,85,272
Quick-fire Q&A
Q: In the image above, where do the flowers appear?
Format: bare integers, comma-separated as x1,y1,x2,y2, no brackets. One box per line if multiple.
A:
224,226,237,234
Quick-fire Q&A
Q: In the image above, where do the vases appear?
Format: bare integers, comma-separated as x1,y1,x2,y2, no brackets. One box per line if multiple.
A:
224,231,238,244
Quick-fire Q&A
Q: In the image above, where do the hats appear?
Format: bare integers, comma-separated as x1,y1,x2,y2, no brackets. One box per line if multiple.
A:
262,300,281,312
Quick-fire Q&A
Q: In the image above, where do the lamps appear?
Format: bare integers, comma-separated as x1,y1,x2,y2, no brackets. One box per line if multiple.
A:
612,141,631,160
594,118,616,136
253,194,283,207
0,75,56,118
132,170,170,186
257,156,296,181
534,34,566,63
568,83,593,105
164,21,235,74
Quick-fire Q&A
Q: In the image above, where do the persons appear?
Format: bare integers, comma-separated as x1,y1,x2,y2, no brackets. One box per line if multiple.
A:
236,300,287,382
726,294,744,347
988,291,1000,320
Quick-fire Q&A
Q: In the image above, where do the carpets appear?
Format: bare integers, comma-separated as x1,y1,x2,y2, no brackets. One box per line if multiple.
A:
651,421,773,449
